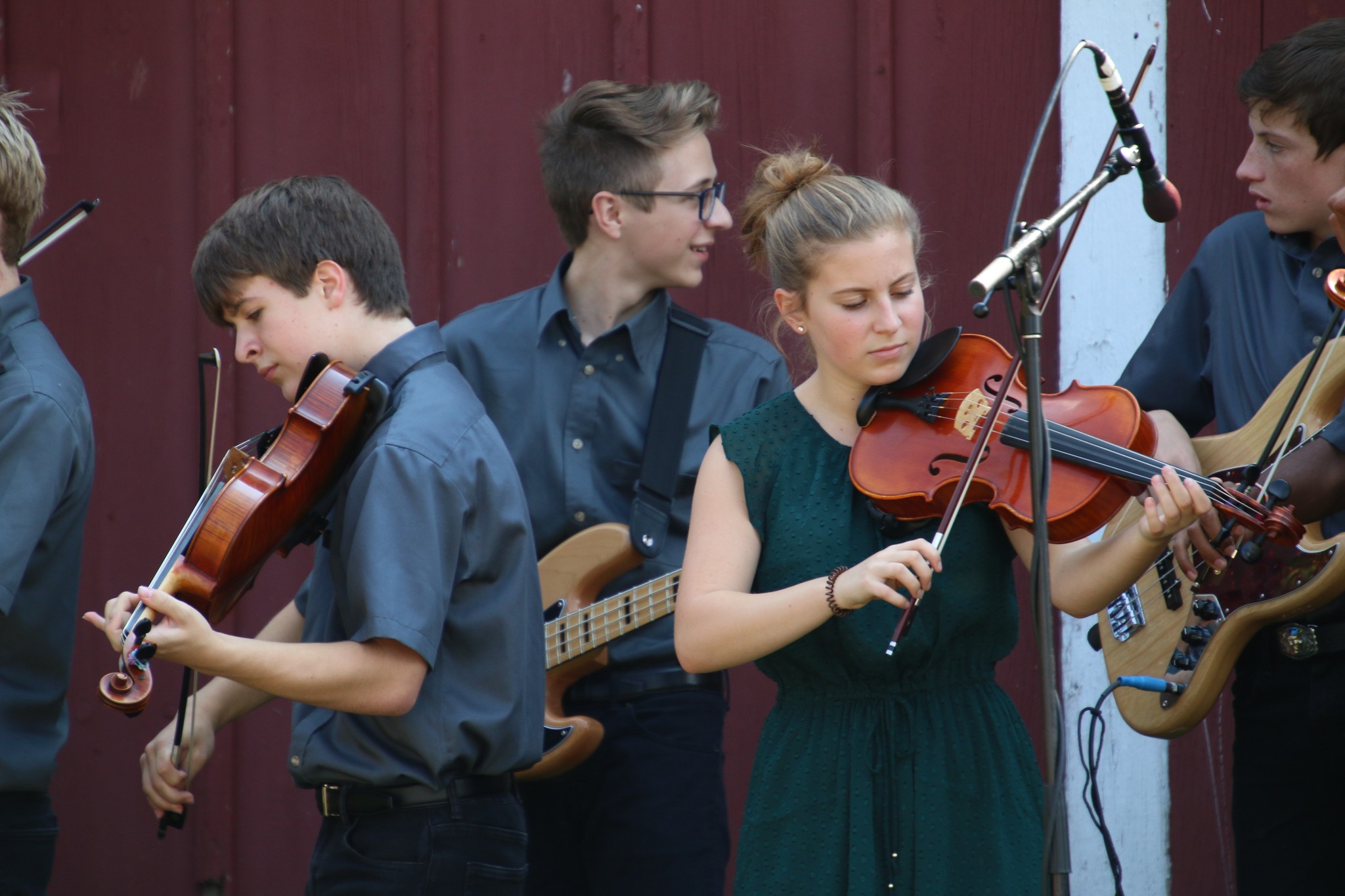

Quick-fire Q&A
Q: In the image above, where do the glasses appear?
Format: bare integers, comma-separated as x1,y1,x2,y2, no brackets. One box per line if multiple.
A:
583,181,728,222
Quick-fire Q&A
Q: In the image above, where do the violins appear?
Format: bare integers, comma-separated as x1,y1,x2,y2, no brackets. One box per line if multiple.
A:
1321,266,1345,311
847,332,1309,548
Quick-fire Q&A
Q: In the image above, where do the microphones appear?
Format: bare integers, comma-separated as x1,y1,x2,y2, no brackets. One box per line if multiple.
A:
1089,45,1182,222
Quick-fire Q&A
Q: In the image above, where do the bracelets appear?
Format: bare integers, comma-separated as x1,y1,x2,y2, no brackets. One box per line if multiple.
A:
826,565,855,617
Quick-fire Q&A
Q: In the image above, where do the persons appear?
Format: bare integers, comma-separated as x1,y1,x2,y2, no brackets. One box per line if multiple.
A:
442,79,792,896
1112,18,1345,896
83,174,544,896
0,87,99,896
672,152,1214,896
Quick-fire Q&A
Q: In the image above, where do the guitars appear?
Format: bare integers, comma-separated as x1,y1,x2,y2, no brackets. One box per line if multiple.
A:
513,521,680,784
97,357,382,722
1099,335,1345,743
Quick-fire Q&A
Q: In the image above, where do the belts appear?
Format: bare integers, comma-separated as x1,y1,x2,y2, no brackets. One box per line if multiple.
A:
578,665,725,704
1266,620,1344,660
316,774,509,816
